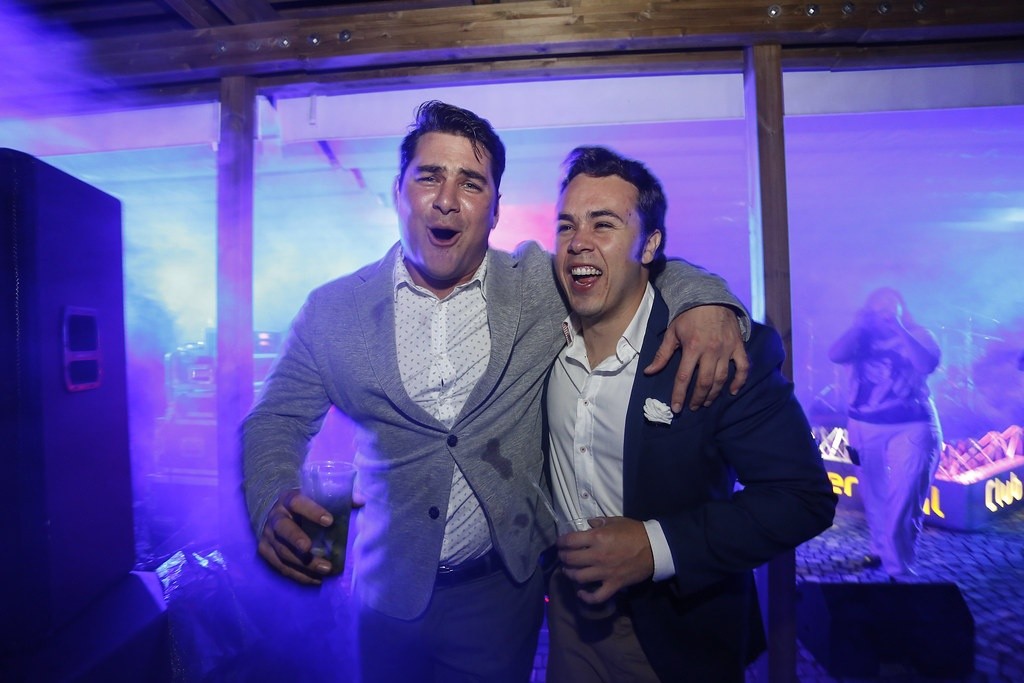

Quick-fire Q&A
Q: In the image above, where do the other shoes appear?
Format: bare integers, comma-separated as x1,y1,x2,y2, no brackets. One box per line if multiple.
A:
861,553,882,569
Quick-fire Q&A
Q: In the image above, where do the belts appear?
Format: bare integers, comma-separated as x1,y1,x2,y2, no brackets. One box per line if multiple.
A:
432,552,506,588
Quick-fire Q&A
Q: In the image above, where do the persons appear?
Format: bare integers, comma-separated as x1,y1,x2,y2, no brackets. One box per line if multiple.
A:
540,144,839,682
827,288,944,581
239,100,752,683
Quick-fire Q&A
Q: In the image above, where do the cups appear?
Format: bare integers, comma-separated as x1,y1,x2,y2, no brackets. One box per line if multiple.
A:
559,518,618,617
299,460,361,577
